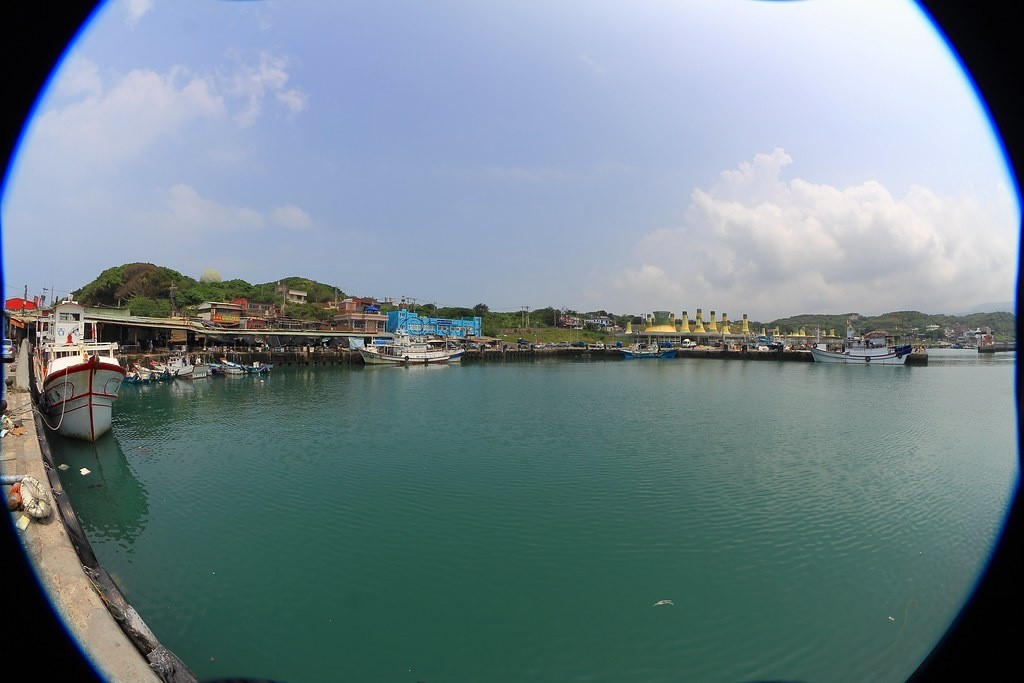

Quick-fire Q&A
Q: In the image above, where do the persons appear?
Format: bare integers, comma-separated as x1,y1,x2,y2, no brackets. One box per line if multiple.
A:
190,356,203,365
95,352,100,362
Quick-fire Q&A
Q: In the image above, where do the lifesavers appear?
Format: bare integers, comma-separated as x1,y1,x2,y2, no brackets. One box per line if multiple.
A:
370,353,374,359
405,356,409,361
866,356,870,362
898,353,902,358
813,343,817,348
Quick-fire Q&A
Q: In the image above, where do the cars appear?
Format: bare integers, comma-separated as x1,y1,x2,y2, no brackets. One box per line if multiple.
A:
481,342,491,348
467,343,477,349
535,343,545,349
638,343,646,348
2,339,15,359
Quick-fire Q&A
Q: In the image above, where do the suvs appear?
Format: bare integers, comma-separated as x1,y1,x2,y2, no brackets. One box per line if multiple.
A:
558,341,570,346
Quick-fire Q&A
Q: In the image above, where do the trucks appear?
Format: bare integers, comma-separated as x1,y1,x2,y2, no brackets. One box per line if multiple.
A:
607,342,624,349
658,341,672,348
682,339,696,348
589,341,603,348
573,341,585,347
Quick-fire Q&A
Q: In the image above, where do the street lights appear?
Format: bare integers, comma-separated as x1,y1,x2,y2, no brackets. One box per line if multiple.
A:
536,322,539,342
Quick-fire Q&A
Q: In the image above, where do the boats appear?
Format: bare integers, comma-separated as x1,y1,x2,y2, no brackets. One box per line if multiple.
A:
126,354,274,383
33,299,126,444
805,342,913,365
355,325,466,364
618,348,680,359
